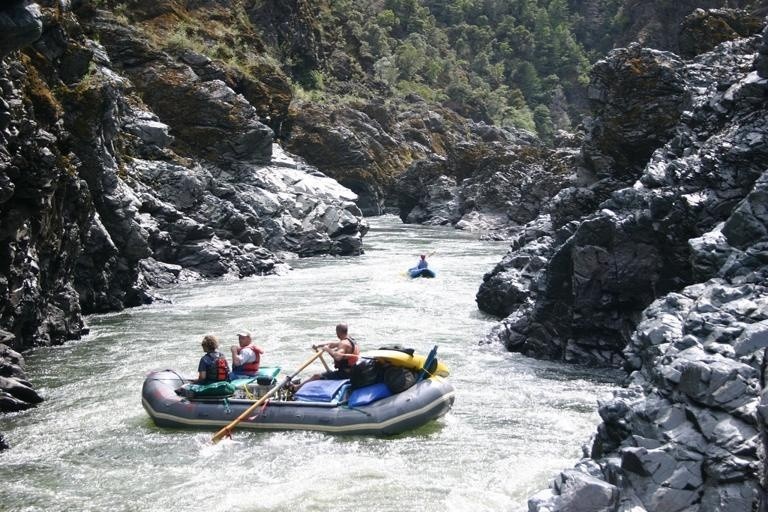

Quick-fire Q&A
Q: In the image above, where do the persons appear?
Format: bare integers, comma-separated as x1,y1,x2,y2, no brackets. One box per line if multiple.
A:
193,334,230,384
287,322,360,393
418,254,428,269
229,330,264,379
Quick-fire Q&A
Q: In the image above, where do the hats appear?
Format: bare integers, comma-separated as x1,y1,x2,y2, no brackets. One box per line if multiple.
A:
236,332,252,337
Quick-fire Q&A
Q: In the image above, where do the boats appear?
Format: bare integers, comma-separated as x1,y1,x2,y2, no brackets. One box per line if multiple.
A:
140,367,455,436
408,266,436,279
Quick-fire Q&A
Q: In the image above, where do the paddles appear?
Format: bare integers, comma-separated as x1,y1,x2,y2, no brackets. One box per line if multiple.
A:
210,344,332,444
